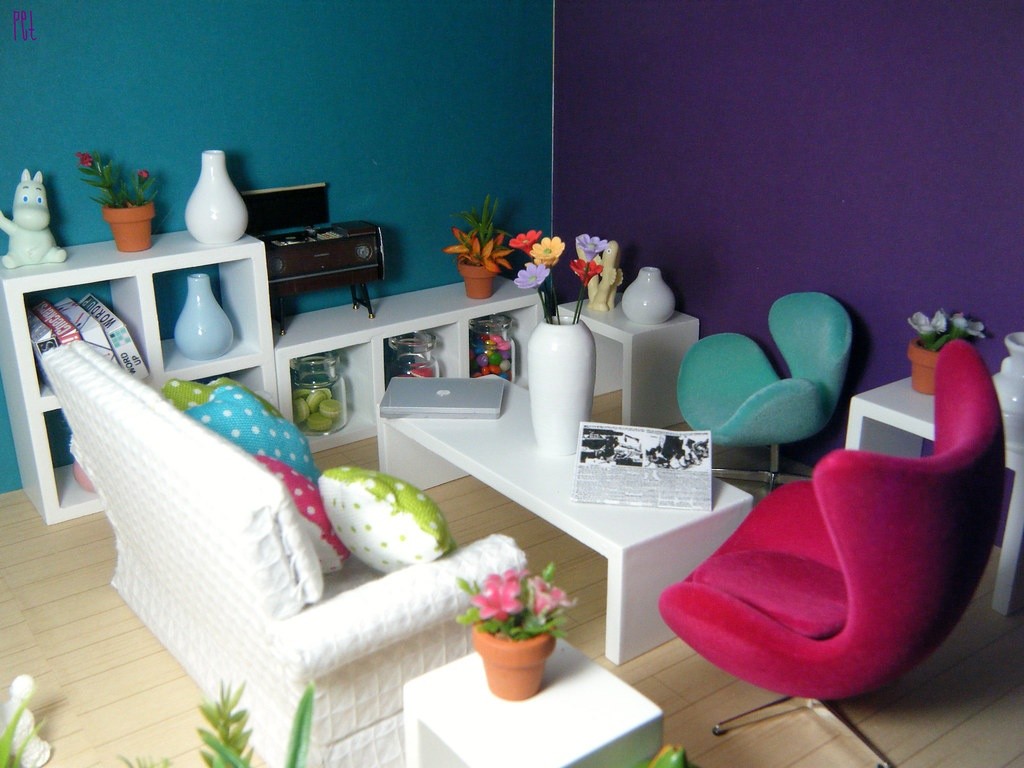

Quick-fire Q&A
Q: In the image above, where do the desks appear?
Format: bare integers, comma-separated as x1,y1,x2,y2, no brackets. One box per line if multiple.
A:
400,637,665,768
376,374,754,665
844,370,1024,614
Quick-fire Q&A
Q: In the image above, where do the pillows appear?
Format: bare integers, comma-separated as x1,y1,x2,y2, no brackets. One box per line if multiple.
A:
318,464,457,572
160,375,348,573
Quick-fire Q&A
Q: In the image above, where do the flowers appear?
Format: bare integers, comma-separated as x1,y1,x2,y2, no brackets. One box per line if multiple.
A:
451,557,575,642
906,308,986,352
507,227,610,323
440,195,515,275
75,151,161,206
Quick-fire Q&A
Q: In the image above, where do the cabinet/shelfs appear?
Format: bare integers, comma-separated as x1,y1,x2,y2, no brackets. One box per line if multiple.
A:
0,227,278,526
272,275,546,452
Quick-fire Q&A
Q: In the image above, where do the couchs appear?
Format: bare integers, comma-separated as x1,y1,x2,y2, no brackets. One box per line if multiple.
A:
35,339,524,767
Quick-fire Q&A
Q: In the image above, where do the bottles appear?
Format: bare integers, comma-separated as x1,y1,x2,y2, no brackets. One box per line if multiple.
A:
468,315,516,385
185,150,249,245
622,267,675,324
386,333,444,392
174,273,233,361
290,348,349,436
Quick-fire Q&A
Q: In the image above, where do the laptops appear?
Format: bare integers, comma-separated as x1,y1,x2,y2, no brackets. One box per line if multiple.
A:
379,376,505,418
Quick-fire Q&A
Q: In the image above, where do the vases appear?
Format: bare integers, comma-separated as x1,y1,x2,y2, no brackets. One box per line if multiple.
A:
458,257,498,298
991,331,1024,444
173,272,234,361
472,618,558,699
907,338,941,396
184,149,248,245
527,315,599,459
622,267,675,325
102,201,155,252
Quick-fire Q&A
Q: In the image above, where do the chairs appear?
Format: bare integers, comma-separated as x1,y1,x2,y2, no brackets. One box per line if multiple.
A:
657,340,1007,767
677,290,854,496
